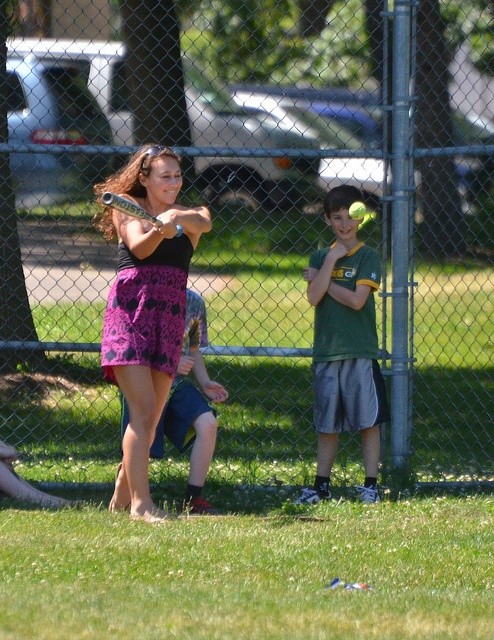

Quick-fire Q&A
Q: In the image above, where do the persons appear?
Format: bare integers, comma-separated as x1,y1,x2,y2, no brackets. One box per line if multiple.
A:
89,142,212,525
107,288,227,516
292,184,391,507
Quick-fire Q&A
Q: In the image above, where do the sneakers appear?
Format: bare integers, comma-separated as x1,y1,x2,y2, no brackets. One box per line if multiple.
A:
180,498,227,515
292,488,333,505
356,484,383,504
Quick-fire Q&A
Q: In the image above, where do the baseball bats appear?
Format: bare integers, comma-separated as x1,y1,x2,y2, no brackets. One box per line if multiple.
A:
100,192,183,237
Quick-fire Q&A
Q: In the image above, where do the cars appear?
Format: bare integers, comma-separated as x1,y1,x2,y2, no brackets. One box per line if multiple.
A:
231,91,425,226
300,102,480,219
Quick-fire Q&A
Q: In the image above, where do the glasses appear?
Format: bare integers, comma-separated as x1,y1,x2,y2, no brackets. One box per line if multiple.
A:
141,147,176,171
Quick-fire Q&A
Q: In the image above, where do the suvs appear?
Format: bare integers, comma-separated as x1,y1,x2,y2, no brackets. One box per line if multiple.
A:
3,54,115,208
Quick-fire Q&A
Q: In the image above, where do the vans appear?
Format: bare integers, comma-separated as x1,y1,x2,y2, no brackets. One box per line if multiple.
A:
4,37,320,212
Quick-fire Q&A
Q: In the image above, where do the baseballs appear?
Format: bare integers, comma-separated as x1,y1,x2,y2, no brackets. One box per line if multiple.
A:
349,201,367,221
